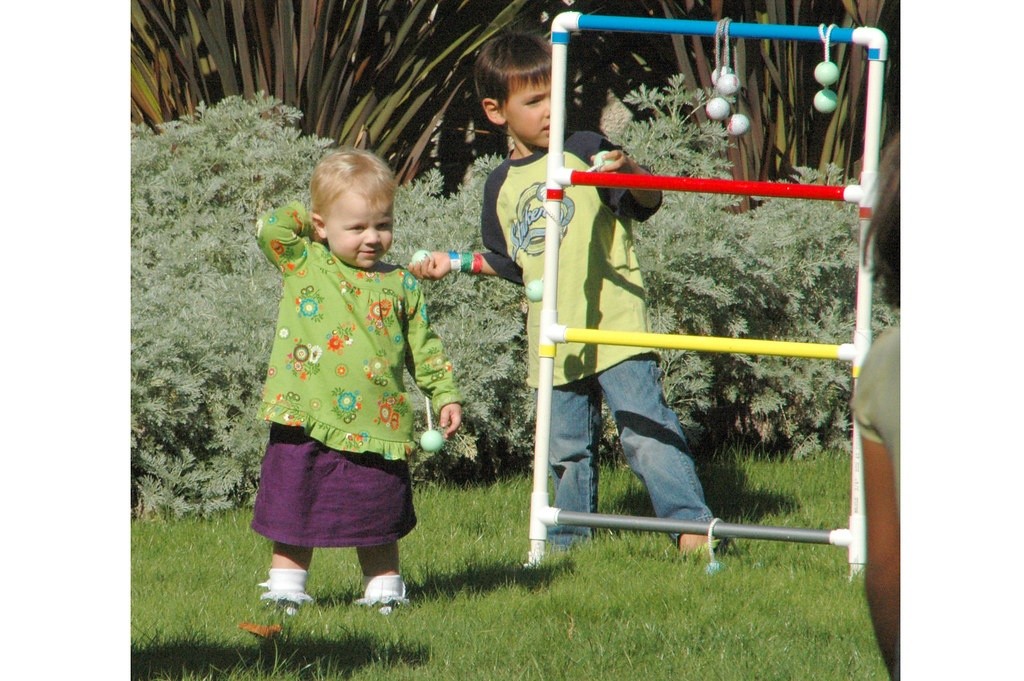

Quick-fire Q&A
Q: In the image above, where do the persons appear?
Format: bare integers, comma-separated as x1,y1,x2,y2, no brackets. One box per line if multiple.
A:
408,25,722,555
849,134,900,681
250,148,464,617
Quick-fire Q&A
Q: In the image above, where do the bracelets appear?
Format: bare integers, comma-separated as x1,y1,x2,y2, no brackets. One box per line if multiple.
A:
448,251,483,276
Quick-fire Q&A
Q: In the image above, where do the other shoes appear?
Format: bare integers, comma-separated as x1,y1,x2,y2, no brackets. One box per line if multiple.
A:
355,597,410,616
676,533,713,553
260,591,313,616
